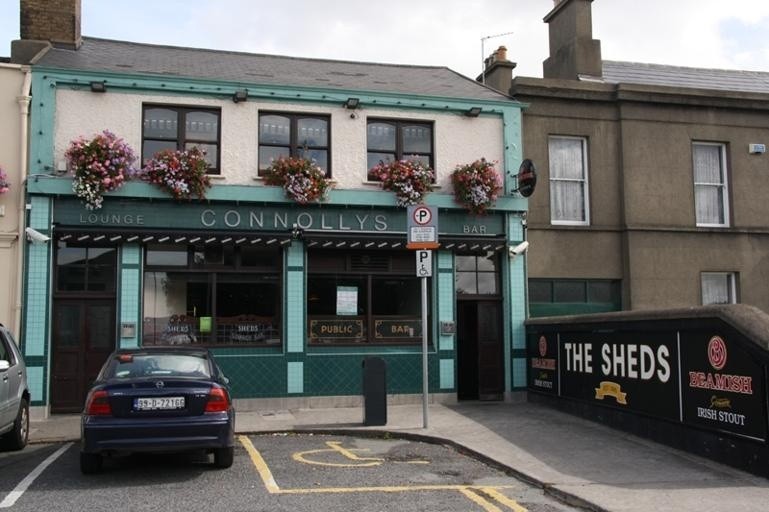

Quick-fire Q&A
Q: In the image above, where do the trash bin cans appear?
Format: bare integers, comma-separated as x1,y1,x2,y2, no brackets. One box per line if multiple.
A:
362,357,387,425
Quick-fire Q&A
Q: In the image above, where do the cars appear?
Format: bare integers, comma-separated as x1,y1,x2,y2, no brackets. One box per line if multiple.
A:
78,345,237,471
0,325,32,451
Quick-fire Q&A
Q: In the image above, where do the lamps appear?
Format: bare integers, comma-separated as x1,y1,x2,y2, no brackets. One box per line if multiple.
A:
91,80,105,93
343,98,359,109
233,89,248,103
465,107,482,117
